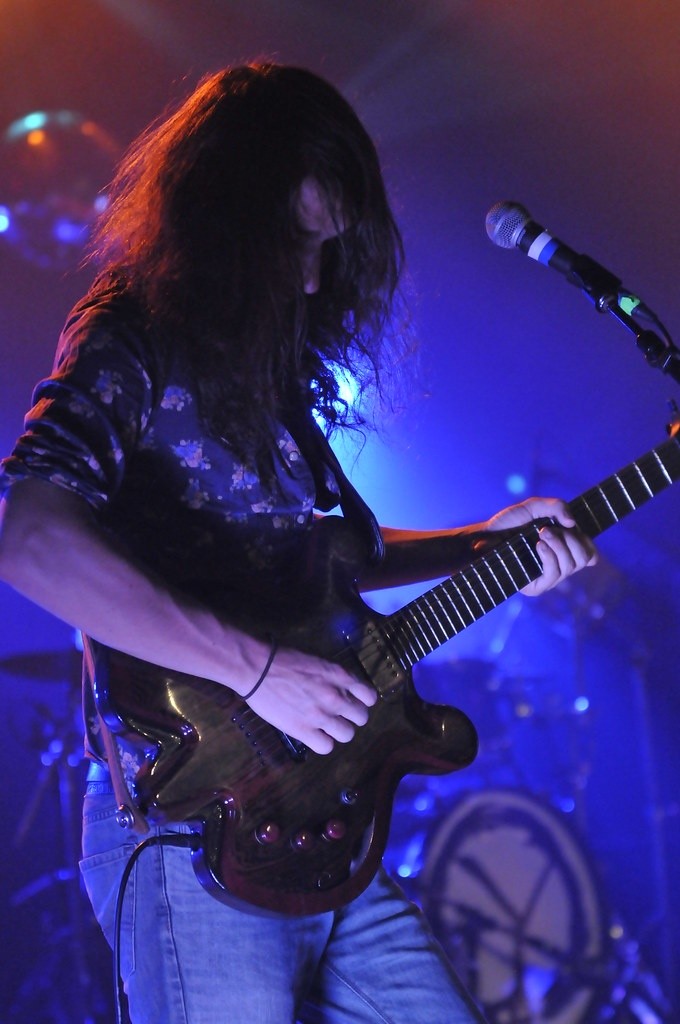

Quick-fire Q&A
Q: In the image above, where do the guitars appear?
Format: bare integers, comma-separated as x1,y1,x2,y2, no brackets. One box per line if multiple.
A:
88,419,680,921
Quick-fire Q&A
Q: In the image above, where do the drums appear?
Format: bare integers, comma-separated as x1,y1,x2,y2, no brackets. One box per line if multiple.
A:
389,787,613,1024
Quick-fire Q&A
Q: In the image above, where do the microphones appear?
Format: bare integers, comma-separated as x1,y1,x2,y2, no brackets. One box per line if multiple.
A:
486,201,659,325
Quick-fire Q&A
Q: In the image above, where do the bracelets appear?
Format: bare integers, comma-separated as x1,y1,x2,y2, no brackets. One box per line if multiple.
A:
234,631,278,701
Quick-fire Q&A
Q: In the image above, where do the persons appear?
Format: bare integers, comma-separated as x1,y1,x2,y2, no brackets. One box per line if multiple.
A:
0,59,602,1024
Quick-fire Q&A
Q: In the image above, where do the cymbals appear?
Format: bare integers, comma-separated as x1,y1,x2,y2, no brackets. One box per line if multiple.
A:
0,649,85,683
